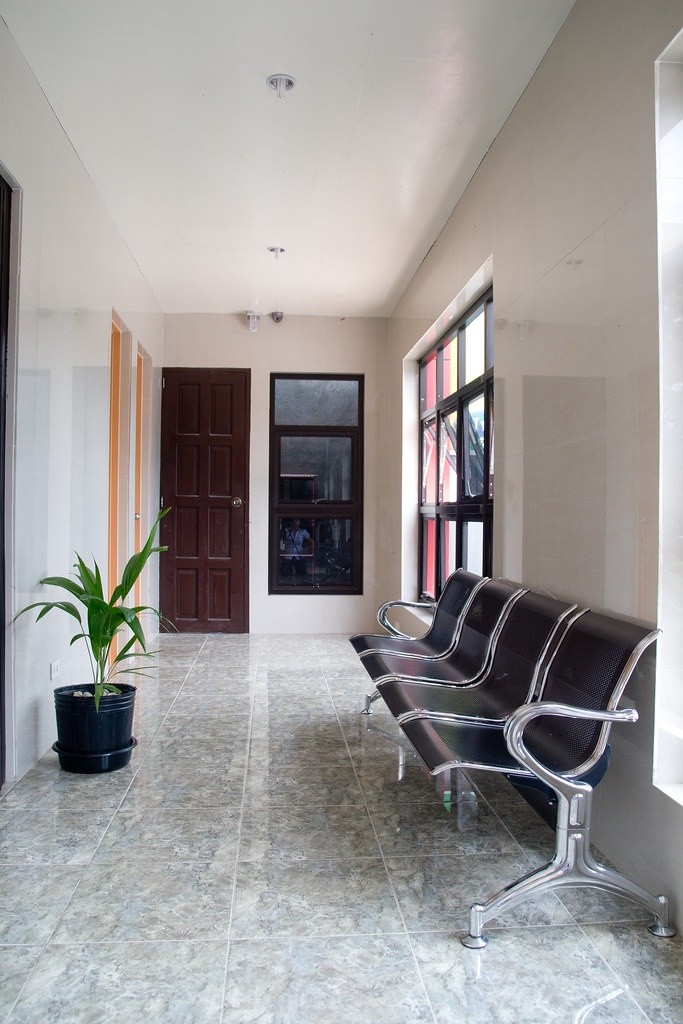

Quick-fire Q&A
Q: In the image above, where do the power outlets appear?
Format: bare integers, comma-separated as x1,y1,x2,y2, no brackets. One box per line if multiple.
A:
50,658,63,680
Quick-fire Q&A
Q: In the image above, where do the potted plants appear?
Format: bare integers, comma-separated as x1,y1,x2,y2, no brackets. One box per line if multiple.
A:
13,506,180,774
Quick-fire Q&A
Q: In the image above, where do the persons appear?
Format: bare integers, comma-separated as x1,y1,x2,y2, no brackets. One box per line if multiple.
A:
281,519,311,574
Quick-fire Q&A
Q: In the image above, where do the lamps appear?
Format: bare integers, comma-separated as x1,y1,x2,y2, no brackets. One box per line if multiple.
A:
265,73,296,102
267,247,285,259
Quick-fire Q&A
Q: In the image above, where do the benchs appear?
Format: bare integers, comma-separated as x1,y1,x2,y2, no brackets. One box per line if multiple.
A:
348,567,677,949
325,540,351,582
